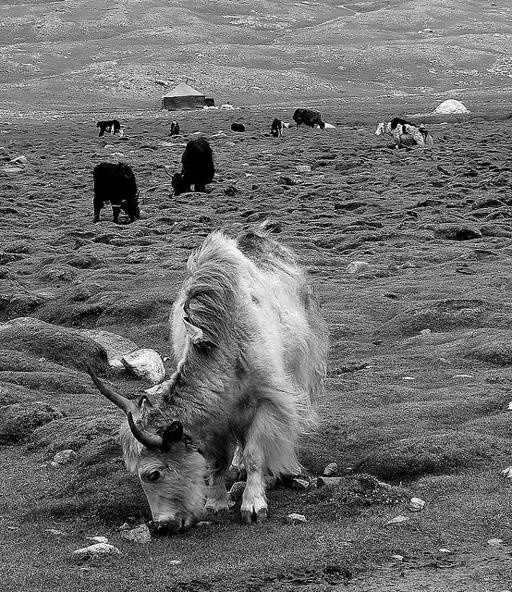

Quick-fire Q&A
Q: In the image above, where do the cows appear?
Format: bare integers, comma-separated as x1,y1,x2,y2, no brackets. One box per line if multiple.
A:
85,220,332,535
374,117,434,149
164,138,215,196
292,108,328,130
96,120,120,137
170,120,179,135
270,119,283,137
230,123,245,132
92,162,141,225
